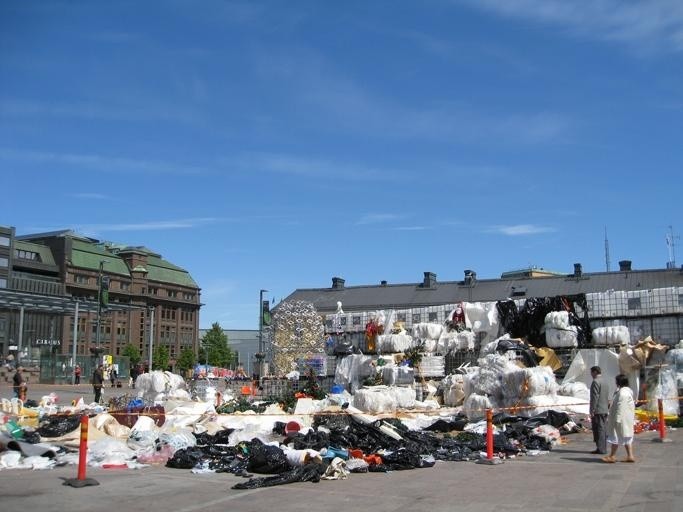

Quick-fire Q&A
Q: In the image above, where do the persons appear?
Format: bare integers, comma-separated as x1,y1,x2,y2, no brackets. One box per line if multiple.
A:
106,357,153,389
12,366,26,400
74,362,81,384
92,364,104,403
598,373,634,463
587,364,610,453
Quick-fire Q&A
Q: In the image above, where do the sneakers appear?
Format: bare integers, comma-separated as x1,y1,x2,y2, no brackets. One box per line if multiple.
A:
620,458,635,462
602,456,616,463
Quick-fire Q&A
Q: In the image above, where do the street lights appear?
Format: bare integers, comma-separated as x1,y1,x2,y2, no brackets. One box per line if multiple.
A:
256,287,272,389
90,258,110,371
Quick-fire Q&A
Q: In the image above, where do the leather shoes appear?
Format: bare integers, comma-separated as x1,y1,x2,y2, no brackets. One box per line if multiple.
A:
590,449,608,454
